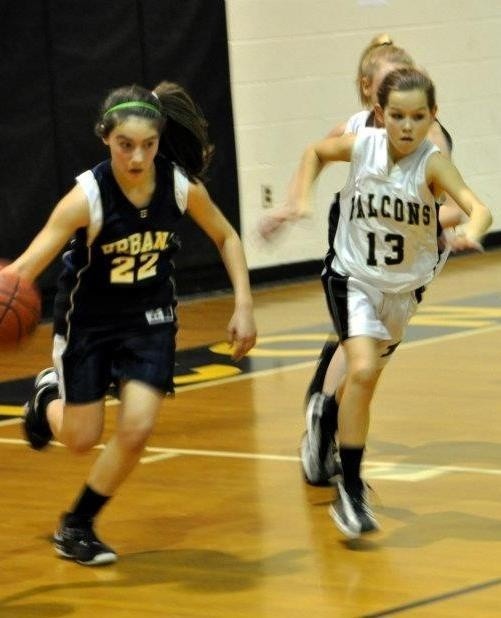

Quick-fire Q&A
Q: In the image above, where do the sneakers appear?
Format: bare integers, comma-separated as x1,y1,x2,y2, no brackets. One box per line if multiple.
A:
52,512,118,567
299,430,340,488
304,392,337,475
327,476,378,539
24,367,60,450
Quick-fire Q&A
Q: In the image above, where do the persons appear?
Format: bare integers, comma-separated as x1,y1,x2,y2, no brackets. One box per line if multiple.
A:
0,79,257,566
257,35,469,484
291,69,492,543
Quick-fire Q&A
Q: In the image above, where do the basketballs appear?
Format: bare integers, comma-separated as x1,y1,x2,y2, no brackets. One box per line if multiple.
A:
0,271,43,346
